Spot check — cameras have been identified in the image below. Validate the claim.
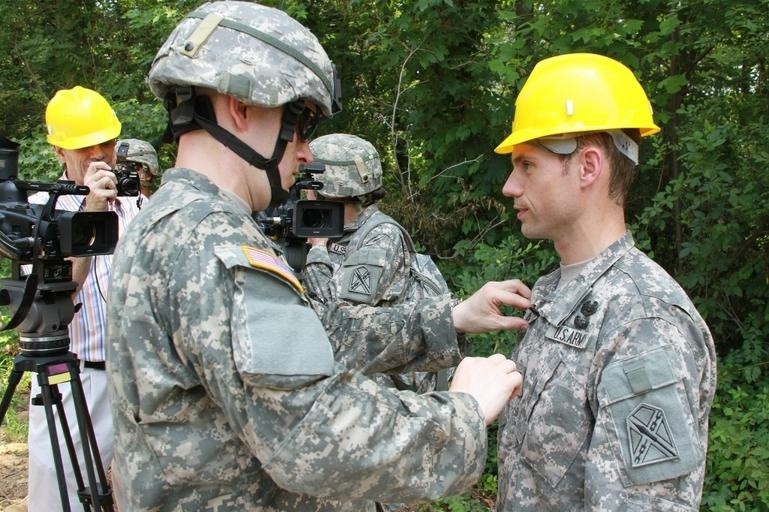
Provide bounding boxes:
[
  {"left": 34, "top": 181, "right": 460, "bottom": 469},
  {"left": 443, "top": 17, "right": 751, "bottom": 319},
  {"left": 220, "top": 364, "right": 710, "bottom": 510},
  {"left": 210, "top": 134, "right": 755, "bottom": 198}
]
[{"left": 112, "top": 170, "right": 141, "bottom": 196}]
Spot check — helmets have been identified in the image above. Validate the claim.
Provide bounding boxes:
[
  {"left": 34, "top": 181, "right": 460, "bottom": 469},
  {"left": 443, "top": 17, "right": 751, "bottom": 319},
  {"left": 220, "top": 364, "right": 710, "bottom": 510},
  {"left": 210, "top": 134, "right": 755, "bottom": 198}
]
[
  {"left": 45, "top": 86, "right": 123, "bottom": 151},
  {"left": 494, "top": 52, "right": 662, "bottom": 155},
  {"left": 148, "top": 1, "right": 335, "bottom": 121},
  {"left": 114, "top": 138, "right": 160, "bottom": 176},
  {"left": 307, "top": 133, "right": 383, "bottom": 199}
]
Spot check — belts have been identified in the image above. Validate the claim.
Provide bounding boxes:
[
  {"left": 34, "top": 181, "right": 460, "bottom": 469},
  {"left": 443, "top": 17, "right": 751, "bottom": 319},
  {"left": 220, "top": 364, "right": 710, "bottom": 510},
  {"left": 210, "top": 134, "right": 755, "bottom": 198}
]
[{"left": 84, "top": 361, "right": 105, "bottom": 368}]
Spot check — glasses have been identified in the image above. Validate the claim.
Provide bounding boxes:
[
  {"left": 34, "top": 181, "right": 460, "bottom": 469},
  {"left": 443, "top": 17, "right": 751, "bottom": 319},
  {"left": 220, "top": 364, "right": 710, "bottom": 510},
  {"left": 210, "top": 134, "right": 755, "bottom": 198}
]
[{"left": 294, "top": 106, "right": 321, "bottom": 143}]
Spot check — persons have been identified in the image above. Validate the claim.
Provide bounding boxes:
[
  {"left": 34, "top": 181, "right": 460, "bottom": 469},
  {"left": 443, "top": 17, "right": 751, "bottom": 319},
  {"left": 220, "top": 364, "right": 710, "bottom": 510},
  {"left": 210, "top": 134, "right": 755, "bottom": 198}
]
[
  {"left": 104, "top": 0, "right": 532, "bottom": 512},
  {"left": 493, "top": 52, "right": 717, "bottom": 512},
  {"left": 115, "top": 139, "right": 158, "bottom": 199},
  {"left": 28, "top": 85, "right": 149, "bottom": 512},
  {"left": 303, "top": 133, "right": 457, "bottom": 395}
]
